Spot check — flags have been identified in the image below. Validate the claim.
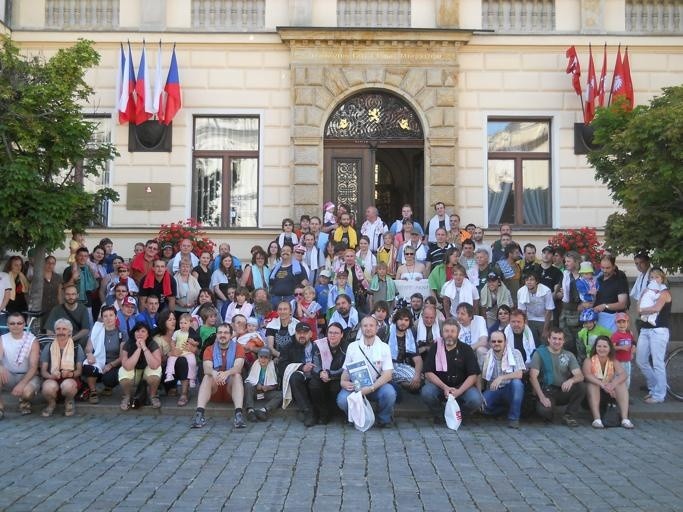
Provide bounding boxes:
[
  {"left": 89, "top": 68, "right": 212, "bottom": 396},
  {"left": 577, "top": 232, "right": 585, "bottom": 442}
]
[
  {"left": 585, "top": 46, "right": 597, "bottom": 127},
  {"left": 117, "top": 41, "right": 128, "bottom": 126},
  {"left": 566, "top": 45, "right": 581, "bottom": 95},
  {"left": 119, "top": 41, "right": 137, "bottom": 123},
  {"left": 611, "top": 47, "right": 634, "bottom": 112},
  {"left": 134, "top": 39, "right": 152, "bottom": 125},
  {"left": 597, "top": 47, "right": 610, "bottom": 107},
  {"left": 160, "top": 42, "right": 182, "bottom": 124},
  {"left": 150, "top": 39, "right": 166, "bottom": 125}
]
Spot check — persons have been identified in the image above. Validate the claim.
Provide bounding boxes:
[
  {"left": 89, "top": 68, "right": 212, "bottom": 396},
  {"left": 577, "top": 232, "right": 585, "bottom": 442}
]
[
  {"left": 575, "top": 261, "right": 603, "bottom": 303},
  {"left": 346, "top": 298, "right": 390, "bottom": 344},
  {"left": 494, "top": 234, "right": 515, "bottom": 262},
  {"left": 446, "top": 214, "right": 471, "bottom": 252},
  {"left": 280, "top": 322, "right": 317, "bottom": 426},
  {"left": 480, "top": 271, "right": 517, "bottom": 329},
  {"left": 314, "top": 270, "right": 334, "bottom": 329},
  {"left": 210, "top": 243, "right": 243, "bottom": 283},
  {"left": 98, "top": 256, "right": 124, "bottom": 304},
  {"left": 0, "top": 271, "right": 12, "bottom": 336},
  {"left": 97, "top": 282, "right": 139, "bottom": 323},
  {"left": 465, "top": 223, "right": 477, "bottom": 235},
  {"left": 40, "top": 318, "right": 86, "bottom": 417},
  {"left": 533, "top": 246, "right": 564, "bottom": 328},
  {"left": 67, "top": 232, "right": 98, "bottom": 281},
  {"left": 106, "top": 264, "right": 139, "bottom": 307},
  {"left": 640, "top": 280, "right": 661, "bottom": 326},
  {"left": 82, "top": 305, "right": 128, "bottom": 404},
  {"left": 224, "top": 287, "right": 255, "bottom": 324},
  {"left": 136, "top": 295, "right": 161, "bottom": 337},
  {"left": 611, "top": 313, "right": 636, "bottom": 406},
  {"left": 457, "top": 239, "right": 478, "bottom": 272},
  {"left": 275, "top": 218, "right": 300, "bottom": 252},
  {"left": 323, "top": 204, "right": 356, "bottom": 233},
  {"left": 517, "top": 271, "right": 555, "bottom": 349},
  {"left": 100, "top": 238, "right": 119, "bottom": 274},
  {"left": 494, "top": 243, "right": 521, "bottom": 309},
  {"left": 173, "top": 256, "right": 202, "bottom": 331},
  {"left": 270, "top": 244, "right": 309, "bottom": 311},
  {"left": 408, "top": 304, "right": 443, "bottom": 394},
  {"left": 577, "top": 308, "right": 613, "bottom": 358},
  {"left": 361, "top": 206, "right": 388, "bottom": 259},
  {"left": 243, "top": 349, "right": 283, "bottom": 422},
  {"left": 630, "top": 254, "right": 653, "bottom": 392},
  {"left": 251, "top": 245, "right": 264, "bottom": 255},
  {"left": 190, "top": 323, "right": 246, "bottom": 428},
  {"left": 491, "top": 223, "right": 523, "bottom": 264},
  {"left": 324, "top": 240, "right": 337, "bottom": 272},
  {"left": 331, "top": 242, "right": 351, "bottom": 274},
  {"left": 192, "top": 252, "right": 212, "bottom": 289},
  {"left": 191, "top": 302, "right": 220, "bottom": 331},
  {"left": 231, "top": 315, "right": 269, "bottom": 353},
  {"left": 393, "top": 217, "right": 424, "bottom": 274},
  {"left": 425, "top": 296, "right": 447, "bottom": 320},
  {"left": 323, "top": 202, "right": 337, "bottom": 237},
  {"left": 515, "top": 243, "right": 544, "bottom": 275},
  {"left": 578, "top": 254, "right": 631, "bottom": 332},
  {"left": 160, "top": 243, "right": 176, "bottom": 265},
  {"left": 457, "top": 302, "right": 488, "bottom": 393},
  {"left": 329, "top": 212, "right": 362, "bottom": 254},
  {"left": 2, "top": 256, "right": 29, "bottom": 331},
  {"left": 237, "top": 317, "right": 264, "bottom": 378},
  {"left": 328, "top": 271, "right": 356, "bottom": 322},
  {"left": 636, "top": 267, "right": 672, "bottom": 403},
  {"left": 354, "top": 235, "right": 377, "bottom": 314},
  {"left": 302, "top": 232, "right": 325, "bottom": 289},
  {"left": 164, "top": 313, "right": 202, "bottom": 388},
  {"left": 293, "top": 245, "right": 310, "bottom": 280},
  {"left": 422, "top": 202, "right": 452, "bottom": 249},
  {"left": 295, "top": 215, "right": 311, "bottom": 243},
  {"left": 44, "top": 285, "right": 89, "bottom": 385},
  {"left": 129, "top": 243, "right": 146, "bottom": 269},
  {"left": 376, "top": 308, "right": 423, "bottom": 394},
  {"left": 472, "top": 227, "right": 493, "bottom": 264},
  {"left": 465, "top": 249, "right": 511, "bottom": 317},
  {"left": 405, "top": 293, "right": 425, "bottom": 333},
  {"left": 366, "top": 261, "right": 399, "bottom": 318},
  {"left": 88, "top": 245, "right": 107, "bottom": 323},
  {"left": 117, "top": 295, "right": 136, "bottom": 343},
  {"left": 425, "top": 227, "right": 456, "bottom": 278},
  {"left": 428, "top": 247, "right": 469, "bottom": 319},
  {"left": 336, "top": 316, "right": 397, "bottom": 428},
  {"left": 153, "top": 311, "right": 200, "bottom": 406},
  {"left": 529, "top": 328, "right": 586, "bottom": 427},
  {"left": 298, "top": 286, "right": 322, "bottom": 342},
  {"left": 440, "top": 265, "right": 480, "bottom": 322},
  {"left": 0, "top": 312, "right": 40, "bottom": 420},
  {"left": 221, "top": 285, "right": 238, "bottom": 322},
  {"left": 265, "top": 300, "right": 301, "bottom": 390},
  {"left": 552, "top": 250, "right": 583, "bottom": 359},
  {"left": 328, "top": 294, "right": 369, "bottom": 336},
  {"left": 487, "top": 304, "right": 512, "bottom": 342},
  {"left": 582, "top": 336, "right": 635, "bottom": 428},
  {"left": 251, "top": 288, "right": 272, "bottom": 348},
  {"left": 390, "top": 203, "right": 424, "bottom": 240},
  {"left": 41, "top": 256, "right": 62, "bottom": 334},
  {"left": 289, "top": 287, "right": 322, "bottom": 341},
  {"left": 395, "top": 246, "right": 427, "bottom": 281},
  {"left": 138, "top": 258, "right": 177, "bottom": 313},
  {"left": 421, "top": 319, "right": 482, "bottom": 424},
  {"left": 502, "top": 309, "right": 542, "bottom": 411},
  {"left": 310, "top": 216, "right": 333, "bottom": 258},
  {"left": 264, "top": 241, "right": 282, "bottom": 272},
  {"left": 165, "top": 239, "right": 200, "bottom": 277},
  {"left": 309, "top": 322, "right": 350, "bottom": 424},
  {"left": 336, "top": 249, "right": 369, "bottom": 309},
  {"left": 189, "top": 288, "right": 224, "bottom": 328},
  {"left": 118, "top": 321, "right": 162, "bottom": 410},
  {"left": 396, "top": 228, "right": 429, "bottom": 272},
  {"left": 24, "top": 248, "right": 36, "bottom": 312},
  {"left": 63, "top": 247, "right": 99, "bottom": 341},
  {"left": 209, "top": 252, "right": 237, "bottom": 315},
  {"left": 240, "top": 251, "right": 271, "bottom": 293},
  {"left": 377, "top": 232, "right": 398, "bottom": 276},
  {"left": 195, "top": 307, "right": 219, "bottom": 384},
  {"left": 132, "top": 240, "right": 160, "bottom": 288},
  {"left": 474, "top": 330, "right": 526, "bottom": 429},
  {"left": 255, "top": 300, "right": 280, "bottom": 325}
]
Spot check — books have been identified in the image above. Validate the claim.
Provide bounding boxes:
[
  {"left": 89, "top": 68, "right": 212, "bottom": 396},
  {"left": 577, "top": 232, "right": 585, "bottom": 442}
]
[{"left": 346, "top": 360, "right": 374, "bottom": 393}]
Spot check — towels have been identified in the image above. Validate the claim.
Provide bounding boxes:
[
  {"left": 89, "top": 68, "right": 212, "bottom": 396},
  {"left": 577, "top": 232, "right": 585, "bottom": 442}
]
[
  {"left": 270, "top": 257, "right": 301, "bottom": 279},
  {"left": 396, "top": 219, "right": 403, "bottom": 233},
  {"left": 142, "top": 309, "right": 154, "bottom": 329},
  {"left": 562, "top": 270, "right": 570, "bottom": 303},
  {"left": 361, "top": 217, "right": 384, "bottom": 235},
  {"left": 346, "top": 391, "right": 365, "bottom": 428},
  {"left": 481, "top": 344, "right": 516, "bottom": 382},
  {"left": 266, "top": 315, "right": 301, "bottom": 336},
  {"left": 325, "top": 255, "right": 331, "bottom": 270},
  {"left": 519, "top": 257, "right": 542, "bottom": 270},
  {"left": 244, "top": 359, "right": 278, "bottom": 386},
  {"left": 224, "top": 301, "right": 252, "bottom": 324},
  {"left": 313, "top": 337, "right": 333, "bottom": 371},
  {"left": 317, "top": 232, "right": 329, "bottom": 252},
  {"left": 416, "top": 312, "right": 441, "bottom": 342},
  {"left": 50, "top": 338, "right": 74, "bottom": 376},
  {"left": 591, "top": 354, "right": 614, "bottom": 385},
  {"left": 143, "top": 268, "right": 172, "bottom": 296},
  {"left": 213, "top": 255, "right": 240, "bottom": 271},
  {"left": 359, "top": 334, "right": 384, "bottom": 361},
  {"left": 377, "top": 244, "right": 397, "bottom": 276},
  {"left": 174, "top": 271, "right": 202, "bottom": 301},
  {"left": 504, "top": 323, "right": 536, "bottom": 364},
  {"left": 78, "top": 265, "right": 99, "bottom": 304},
  {"left": 16, "top": 331, "right": 34, "bottom": 366},
  {"left": 187, "top": 327, "right": 202, "bottom": 349},
  {"left": 435, "top": 337, "right": 447, "bottom": 372},
  {"left": 213, "top": 337, "right": 237, "bottom": 383},
  {"left": 467, "top": 264, "right": 479, "bottom": 286},
  {"left": 87, "top": 261, "right": 107, "bottom": 279},
  {"left": 428, "top": 213, "right": 451, "bottom": 244},
  {"left": 209, "top": 268, "right": 228, "bottom": 292},
  {"left": 444, "top": 278, "right": 473, "bottom": 306},
  {"left": 303, "top": 301, "right": 322, "bottom": 318},
  {"left": 629, "top": 266, "right": 651, "bottom": 300},
  {"left": 428, "top": 264, "right": 446, "bottom": 295},
  {"left": 481, "top": 281, "right": 509, "bottom": 309},
  {"left": 328, "top": 306, "right": 358, "bottom": 330},
  {"left": 327, "top": 282, "right": 355, "bottom": 309},
  {"left": 9, "top": 272, "right": 29, "bottom": 301},
  {"left": 133, "top": 251, "right": 159, "bottom": 275},
  {"left": 332, "top": 256, "right": 340, "bottom": 273},
  {"left": 237, "top": 332, "right": 266, "bottom": 346},
  {"left": 488, "top": 319, "right": 499, "bottom": 341},
  {"left": 471, "top": 319, "right": 479, "bottom": 344},
  {"left": 311, "top": 246, "right": 318, "bottom": 270},
  {"left": 173, "top": 251, "right": 199, "bottom": 273},
  {"left": 289, "top": 299, "right": 297, "bottom": 316},
  {"left": 370, "top": 273, "right": 395, "bottom": 302},
  {"left": 251, "top": 264, "right": 270, "bottom": 291},
  {"left": 460, "top": 229, "right": 472, "bottom": 242},
  {"left": 388, "top": 322, "right": 416, "bottom": 361},
  {"left": 117, "top": 310, "right": 135, "bottom": 332},
  {"left": 279, "top": 232, "right": 298, "bottom": 248},
  {"left": 282, "top": 362, "right": 303, "bottom": 410},
  {"left": 339, "top": 263, "right": 369, "bottom": 290},
  {"left": 333, "top": 226, "right": 357, "bottom": 249},
  {"left": 83, "top": 322, "right": 106, "bottom": 373},
  {"left": 460, "top": 256, "right": 469, "bottom": 270},
  {"left": 496, "top": 259, "right": 516, "bottom": 279},
  {"left": 355, "top": 249, "right": 372, "bottom": 274},
  {"left": 519, "top": 283, "right": 547, "bottom": 304},
  {"left": 530, "top": 344, "right": 554, "bottom": 385},
  {"left": 402, "top": 238, "right": 426, "bottom": 264}
]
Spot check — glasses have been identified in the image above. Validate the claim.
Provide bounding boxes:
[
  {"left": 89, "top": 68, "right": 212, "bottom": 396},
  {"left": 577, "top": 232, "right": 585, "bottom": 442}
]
[
  {"left": 411, "top": 233, "right": 419, "bottom": 236},
  {"left": 116, "top": 290, "right": 128, "bottom": 292},
  {"left": 492, "top": 340, "right": 503, "bottom": 343},
  {"left": 404, "top": 252, "right": 414, "bottom": 255},
  {"left": 294, "top": 293, "right": 302, "bottom": 297},
  {"left": 217, "top": 331, "right": 231, "bottom": 334},
  {"left": 284, "top": 223, "right": 292, "bottom": 226},
  {"left": 100, "top": 305, "right": 113, "bottom": 310},
  {"left": 10, "top": 321, "right": 24, "bottom": 325},
  {"left": 296, "top": 250, "right": 304, "bottom": 255},
  {"left": 47, "top": 262, "right": 55, "bottom": 265},
  {"left": 148, "top": 247, "right": 158, "bottom": 250},
  {"left": 498, "top": 312, "right": 509, "bottom": 315},
  {"left": 119, "top": 270, "right": 127, "bottom": 273},
  {"left": 566, "top": 253, "right": 575, "bottom": 257}
]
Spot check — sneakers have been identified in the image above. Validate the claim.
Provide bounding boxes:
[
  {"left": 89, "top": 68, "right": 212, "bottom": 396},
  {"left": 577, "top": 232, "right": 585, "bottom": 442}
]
[
  {"left": 190, "top": 411, "right": 207, "bottom": 428},
  {"left": 233, "top": 412, "right": 247, "bottom": 428},
  {"left": 296, "top": 410, "right": 305, "bottom": 423},
  {"left": 101, "top": 387, "right": 112, "bottom": 396},
  {"left": 508, "top": 419, "right": 520, "bottom": 429},
  {"left": 621, "top": 422, "right": 634, "bottom": 429},
  {"left": 168, "top": 391, "right": 178, "bottom": 397},
  {"left": 377, "top": 422, "right": 393, "bottom": 428},
  {"left": 164, "top": 375, "right": 174, "bottom": 382},
  {"left": 544, "top": 413, "right": 554, "bottom": 424},
  {"left": 496, "top": 414, "right": 506, "bottom": 420},
  {"left": 149, "top": 395, "right": 161, "bottom": 409},
  {"left": 246, "top": 411, "right": 258, "bottom": 422},
  {"left": 317, "top": 416, "right": 331, "bottom": 425},
  {"left": 561, "top": 414, "right": 578, "bottom": 427},
  {"left": 304, "top": 415, "right": 317, "bottom": 427},
  {"left": 434, "top": 416, "right": 446, "bottom": 425},
  {"left": 120, "top": 396, "right": 130, "bottom": 410},
  {"left": 89, "top": 391, "right": 99, "bottom": 404},
  {"left": 592, "top": 421, "right": 604, "bottom": 429},
  {"left": 256, "top": 409, "right": 268, "bottom": 422},
  {"left": 190, "top": 381, "right": 196, "bottom": 388},
  {"left": 177, "top": 398, "right": 189, "bottom": 406}
]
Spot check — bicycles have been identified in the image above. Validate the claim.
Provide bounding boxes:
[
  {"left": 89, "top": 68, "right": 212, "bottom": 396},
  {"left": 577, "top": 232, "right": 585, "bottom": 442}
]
[
  {"left": 1, "top": 311, "right": 83, "bottom": 387},
  {"left": 666, "top": 345, "right": 683, "bottom": 402}
]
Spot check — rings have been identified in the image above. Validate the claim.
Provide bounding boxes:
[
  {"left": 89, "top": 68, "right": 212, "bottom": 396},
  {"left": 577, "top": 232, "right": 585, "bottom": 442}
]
[{"left": 89, "top": 360, "right": 90, "bottom": 362}]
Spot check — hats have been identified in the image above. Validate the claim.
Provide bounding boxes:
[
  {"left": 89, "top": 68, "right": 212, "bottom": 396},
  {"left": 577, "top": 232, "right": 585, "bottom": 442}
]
[
  {"left": 486, "top": 271, "right": 498, "bottom": 282},
  {"left": 163, "top": 242, "right": 173, "bottom": 249},
  {"left": 294, "top": 245, "right": 306, "bottom": 252},
  {"left": 615, "top": 312, "right": 629, "bottom": 322},
  {"left": 410, "top": 228, "right": 421, "bottom": 235},
  {"left": 323, "top": 202, "right": 335, "bottom": 210},
  {"left": 258, "top": 347, "right": 272, "bottom": 359},
  {"left": 578, "top": 261, "right": 594, "bottom": 273},
  {"left": 295, "top": 322, "right": 312, "bottom": 332},
  {"left": 410, "top": 293, "right": 423, "bottom": 299},
  {"left": 116, "top": 263, "right": 130, "bottom": 269},
  {"left": 247, "top": 317, "right": 258, "bottom": 326},
  {"left": 647, "top": 280, "right": 660, "bottom": 291},
  {"left": 121, "top": 297, "right": 137, "bottom": 306},
  {"left": 542, "top": 246, "right": 555, "bottom": 255},
  {"left": 318, "top": 269, "right": 332, "bottom": 278}
]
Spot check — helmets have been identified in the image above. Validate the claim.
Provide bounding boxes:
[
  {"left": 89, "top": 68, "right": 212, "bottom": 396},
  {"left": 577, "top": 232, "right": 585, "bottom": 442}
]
[{"left": 580, "top": 308, "right": 598, "bottom": 321}]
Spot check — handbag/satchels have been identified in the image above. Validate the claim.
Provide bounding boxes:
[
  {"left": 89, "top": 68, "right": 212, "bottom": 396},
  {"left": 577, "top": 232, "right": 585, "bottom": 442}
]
[
  {"left": 358, "top": 345, "right": 404, "bottom": 404},
  {"left": 74, "top": 340, "right": 90, "bottom": 402},
  {"left": 563, "top": 314, "right": 580, "bottom": 327},
  {"left": 129, "top": 368, "right": 147, "bottom": 410}
]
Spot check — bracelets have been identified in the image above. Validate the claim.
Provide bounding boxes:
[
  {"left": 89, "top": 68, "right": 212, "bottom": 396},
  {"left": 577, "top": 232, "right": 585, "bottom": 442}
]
[
  {"left": 72, "top": 372, "right": 74, "bottom": 377},
  {"left": 86, "top": 354, "right": 92, "bottom": 355},
  {"left": 440, "top": 384, "right": 445, "bottom": 388},
  {"left": 71, "top": 277, "right": 76, "bottom": 281},
  {"left": 143, "top": 347, "right": 148, "bottom": 352},
  {"left": 369, "top": 386, "right": 375, "bottom": 392},
  {"left": 109, "top": 363, "right": 114, "bottom": 368}
]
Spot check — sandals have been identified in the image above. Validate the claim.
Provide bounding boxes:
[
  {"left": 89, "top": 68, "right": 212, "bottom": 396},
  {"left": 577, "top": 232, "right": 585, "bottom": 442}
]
[
  {"left": 645, "top": 398, "right": 659, "bottom": 403},
  {"left": 0, "top": 408, "right": 5, "bottom": 421},
  {"left": 644, "top": 395, "right": 651, "bottom": 400},
  {"left": 19, "top": 400, "right": 32, "bottom": 416},
  {"left": 41, "top": 405, "right": 56, "bottom": 418},
  {"left": 64, "top": 399, "right": 76, "bottom": 417}
]
